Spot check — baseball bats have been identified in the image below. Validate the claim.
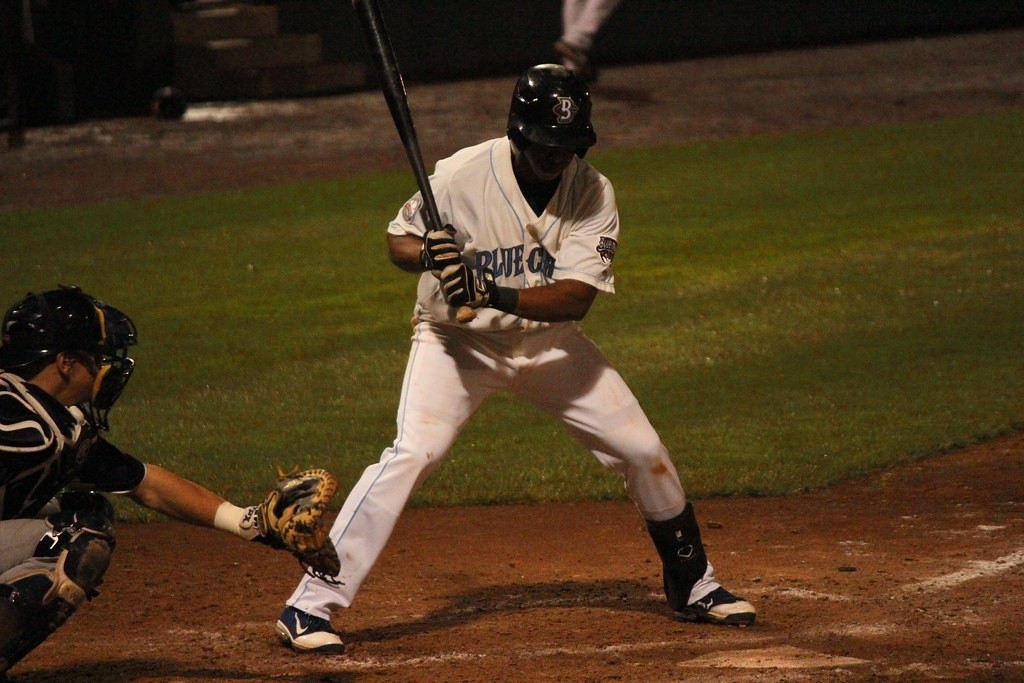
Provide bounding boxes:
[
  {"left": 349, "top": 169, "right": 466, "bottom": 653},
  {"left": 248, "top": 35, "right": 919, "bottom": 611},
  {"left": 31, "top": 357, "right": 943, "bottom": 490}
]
[{"left": 358, "top": 0, "right": 476, "bottom": 325}]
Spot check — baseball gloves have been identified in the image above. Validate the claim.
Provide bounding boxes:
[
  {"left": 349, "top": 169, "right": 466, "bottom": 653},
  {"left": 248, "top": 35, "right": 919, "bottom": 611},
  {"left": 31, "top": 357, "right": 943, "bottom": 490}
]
[{"left": 256, "top": 470, "right": 341, "bottom": 576}]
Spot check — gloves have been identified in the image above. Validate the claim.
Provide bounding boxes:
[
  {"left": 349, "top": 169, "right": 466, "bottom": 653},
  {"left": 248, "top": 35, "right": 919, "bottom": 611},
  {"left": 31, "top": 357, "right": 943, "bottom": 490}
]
[
  {"left": 431, "top": 264, "right": 498, "bottom": 309},
  {"left": 419, "top": 224, "right": 461, "bottom": 268}
]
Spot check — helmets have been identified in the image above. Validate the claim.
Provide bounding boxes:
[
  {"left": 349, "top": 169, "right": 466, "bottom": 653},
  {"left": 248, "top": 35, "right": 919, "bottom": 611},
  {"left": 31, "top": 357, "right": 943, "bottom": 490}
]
[
  {"left": 506, "top": 64, "right": 598, "bottom": 160},
  {"left": 0, "top": 283, "right": 138, "bottom": 373}
]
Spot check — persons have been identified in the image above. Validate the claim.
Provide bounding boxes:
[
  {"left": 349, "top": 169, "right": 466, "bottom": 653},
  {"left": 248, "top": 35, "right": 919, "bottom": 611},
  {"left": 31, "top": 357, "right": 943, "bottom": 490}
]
[
  {"left": 271, "top": 64, "right": 760, "bottom": 655},
  {"left": 0, "top": 284, "right": 344, "bottom": 682}
]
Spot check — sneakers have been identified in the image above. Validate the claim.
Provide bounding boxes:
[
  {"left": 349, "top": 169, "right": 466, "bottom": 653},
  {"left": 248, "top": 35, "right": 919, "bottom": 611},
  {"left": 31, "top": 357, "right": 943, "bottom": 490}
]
[
  {"left": 672, "top": 586, "right": 757, "bottom": 624},
  {"left": 276, "top": 605, "right": 345, "bottom": 654}
]
